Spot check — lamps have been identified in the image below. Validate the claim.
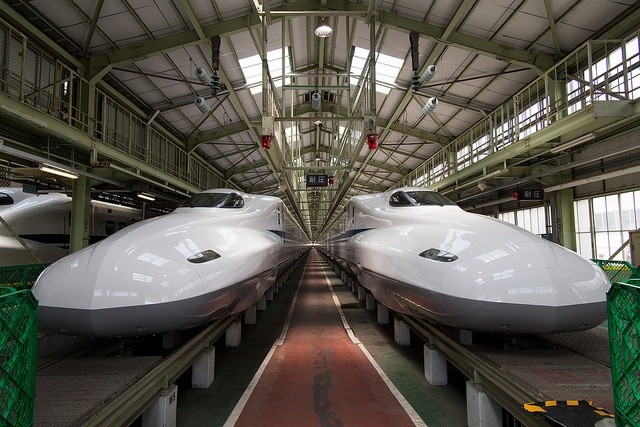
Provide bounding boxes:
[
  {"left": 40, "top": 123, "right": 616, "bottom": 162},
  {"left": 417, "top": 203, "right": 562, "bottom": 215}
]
[{"left": 314, "top": 19, "right": 334, "bottom": 39}]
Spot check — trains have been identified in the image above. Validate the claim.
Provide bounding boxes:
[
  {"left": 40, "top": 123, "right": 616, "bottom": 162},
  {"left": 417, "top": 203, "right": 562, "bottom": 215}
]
[
  {"left": 31, "top": 187, "right": 313, "bottom": 336},
  {"left": 0, "top": 181, "right": 168, "bottom": 267},
  {"left": 313, "top": 187, "right": 612, "bottom": 337}
]
